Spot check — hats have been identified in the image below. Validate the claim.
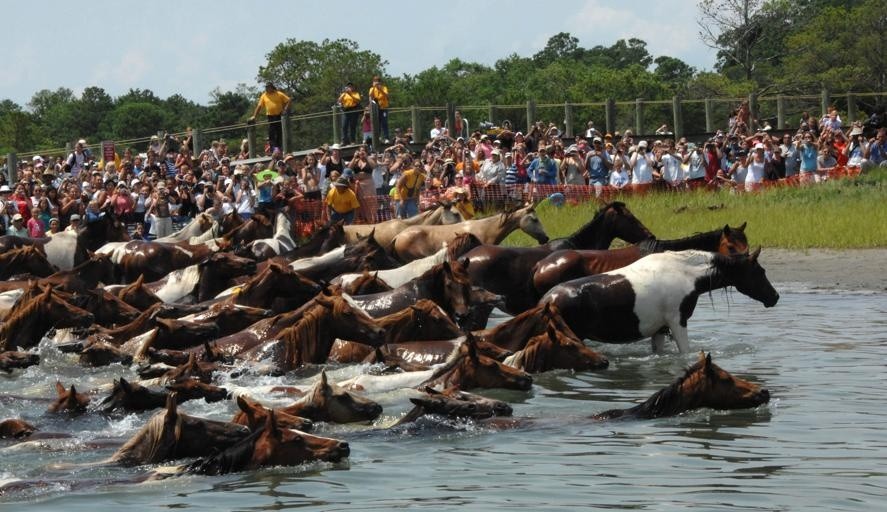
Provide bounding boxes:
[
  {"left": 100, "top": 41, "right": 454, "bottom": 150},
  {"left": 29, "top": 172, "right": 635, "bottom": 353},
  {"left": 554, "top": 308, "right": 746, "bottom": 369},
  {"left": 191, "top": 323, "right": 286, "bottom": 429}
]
[
  {"left": 0, "top": 128, "right": 387, "bottom": 228},
  {"left": 444, "top": 112, "right": 877, "bottom": 184}
]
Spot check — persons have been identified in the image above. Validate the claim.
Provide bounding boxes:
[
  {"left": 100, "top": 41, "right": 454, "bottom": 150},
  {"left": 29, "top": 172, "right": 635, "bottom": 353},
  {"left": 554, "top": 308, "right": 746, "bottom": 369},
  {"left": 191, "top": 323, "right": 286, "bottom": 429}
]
[
  {"left": 337, "top": 82, "right": 362, "bottom": 146},
  {"left": 466, "top": 106, "right": 887, "bottom": 206},
  {"left": 368, "top": 76, "right": 390, "bottom": 145},
  {"left": 247, "top": 82, "right": 292, "bottom": 154},
  {"left": 453, "top": 110, "right": 470, "bottom": 137},
  {"left": 1, "top": 119, "right": 477, "bottom": 240}
]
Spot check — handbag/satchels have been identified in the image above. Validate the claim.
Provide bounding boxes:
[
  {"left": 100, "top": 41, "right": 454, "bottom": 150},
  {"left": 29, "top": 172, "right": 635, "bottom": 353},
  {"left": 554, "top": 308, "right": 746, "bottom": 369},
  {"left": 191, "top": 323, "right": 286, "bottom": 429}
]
[{"left": 407, "top": 187, "right": 414, "bottom": 196}]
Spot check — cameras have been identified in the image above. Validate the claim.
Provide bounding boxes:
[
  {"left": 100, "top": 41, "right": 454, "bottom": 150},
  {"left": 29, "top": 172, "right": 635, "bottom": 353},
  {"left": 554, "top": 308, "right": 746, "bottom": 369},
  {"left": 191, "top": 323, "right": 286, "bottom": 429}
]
[
  {"left": 76, "top": 199, "right": 81, "bottom": 204},
  {"left": 120, "top": 188, "right": 125, "bottom": 193},
  {"left": 355, "top": 151, "right": 363, "bottom": 156},
  {"left": 801, "top": 140, "right": 810, "bottom": 144},
  {"left": 306, "top": 166, "right": 311, "bottom": 170},
  {"left": 751, "top": 148, "right": 756, "bottom": 153}
]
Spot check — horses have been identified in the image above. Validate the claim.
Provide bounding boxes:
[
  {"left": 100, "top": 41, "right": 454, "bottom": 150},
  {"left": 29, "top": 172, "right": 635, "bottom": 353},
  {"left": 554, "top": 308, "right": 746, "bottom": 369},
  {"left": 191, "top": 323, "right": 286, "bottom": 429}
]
[{"left": 0, "top": 198, "right": 781, "bottom": 495}]
